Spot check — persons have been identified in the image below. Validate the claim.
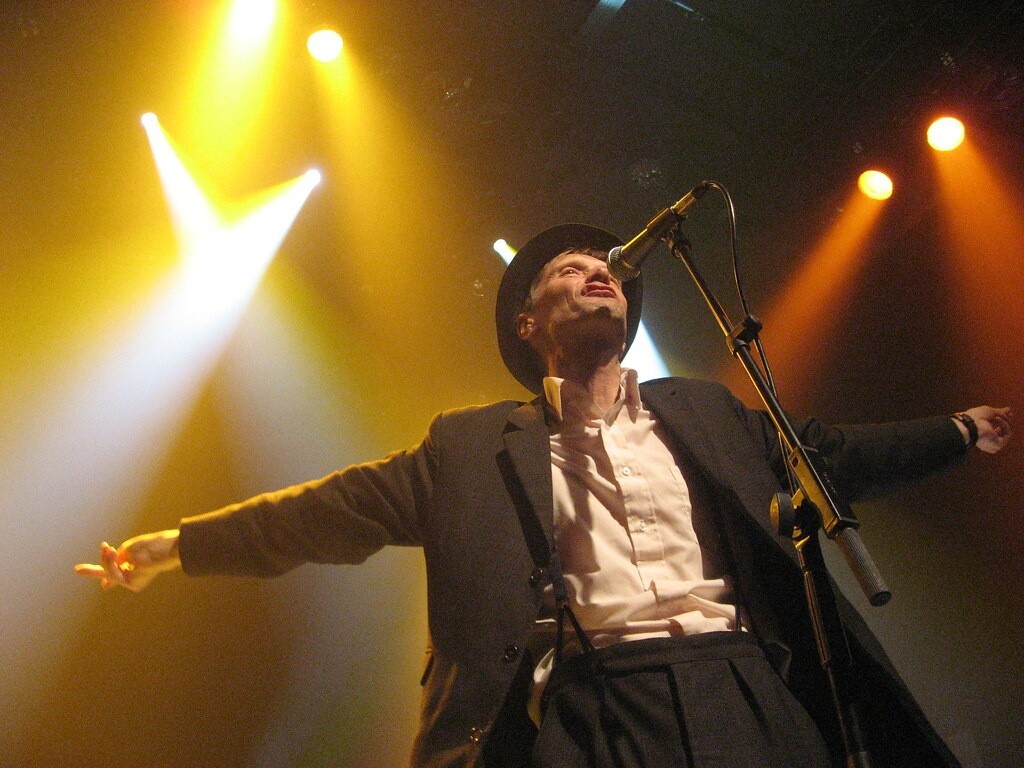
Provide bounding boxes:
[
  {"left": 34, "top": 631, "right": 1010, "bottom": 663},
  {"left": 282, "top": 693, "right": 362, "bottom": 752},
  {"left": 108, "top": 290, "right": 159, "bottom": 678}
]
[{"left": 74, "top": 224, "right": 1024, "bottom": 768}]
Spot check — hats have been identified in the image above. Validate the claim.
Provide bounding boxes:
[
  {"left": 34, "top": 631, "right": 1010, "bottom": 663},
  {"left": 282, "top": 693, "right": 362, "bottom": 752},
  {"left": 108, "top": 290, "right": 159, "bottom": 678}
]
[{"left": 495, "top": 221, "right": 643, "bottom": 396}]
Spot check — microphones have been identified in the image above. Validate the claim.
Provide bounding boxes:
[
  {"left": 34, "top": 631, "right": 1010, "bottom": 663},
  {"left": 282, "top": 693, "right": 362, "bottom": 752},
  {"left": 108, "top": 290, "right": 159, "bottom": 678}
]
[{"left": 606, "top": 178, "right": 712, "bottom": 282}]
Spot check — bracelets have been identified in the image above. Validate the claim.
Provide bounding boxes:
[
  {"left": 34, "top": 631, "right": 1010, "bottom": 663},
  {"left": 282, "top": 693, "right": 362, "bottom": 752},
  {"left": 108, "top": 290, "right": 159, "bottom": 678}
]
[{"left": 949, "top": 413, "right": 979, "bottom": 450}]
